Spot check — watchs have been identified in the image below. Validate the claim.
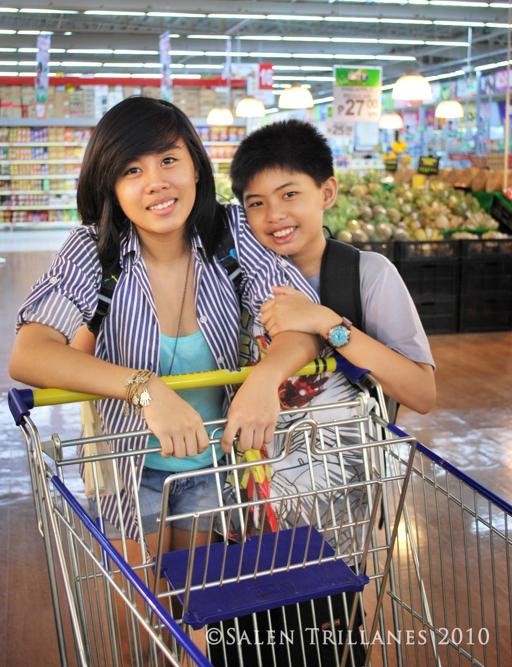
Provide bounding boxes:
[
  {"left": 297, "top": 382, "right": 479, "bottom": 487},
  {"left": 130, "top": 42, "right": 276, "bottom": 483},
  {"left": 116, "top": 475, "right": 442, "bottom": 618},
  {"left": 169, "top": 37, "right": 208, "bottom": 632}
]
[{"left": 327, "top": 313, "right": 353, "bottom": 352}]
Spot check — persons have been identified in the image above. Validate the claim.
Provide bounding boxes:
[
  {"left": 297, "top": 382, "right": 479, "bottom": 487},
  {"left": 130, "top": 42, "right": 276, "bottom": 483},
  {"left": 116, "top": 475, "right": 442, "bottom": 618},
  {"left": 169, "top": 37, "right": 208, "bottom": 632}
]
[
  {"left": 9, "top": 92, "right": 320, "bottom": 666},
  {"left": 69, "top": 116, "right": 439, "bottom": 666}
]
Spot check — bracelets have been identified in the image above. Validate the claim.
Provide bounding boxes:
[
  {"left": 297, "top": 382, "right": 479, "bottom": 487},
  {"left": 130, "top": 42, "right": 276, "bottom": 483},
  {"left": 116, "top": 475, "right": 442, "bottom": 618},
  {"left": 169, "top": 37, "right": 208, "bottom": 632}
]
[{"left": 123, "top": 368, "right": 154, "bottom": 412}]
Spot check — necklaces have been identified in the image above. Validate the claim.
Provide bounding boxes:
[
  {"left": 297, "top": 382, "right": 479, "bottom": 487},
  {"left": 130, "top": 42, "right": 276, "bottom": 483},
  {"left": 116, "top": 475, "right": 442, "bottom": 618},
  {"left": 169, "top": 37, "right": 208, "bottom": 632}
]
[{"left": 160, "top": 251, "right": 191, "bottom": 379}]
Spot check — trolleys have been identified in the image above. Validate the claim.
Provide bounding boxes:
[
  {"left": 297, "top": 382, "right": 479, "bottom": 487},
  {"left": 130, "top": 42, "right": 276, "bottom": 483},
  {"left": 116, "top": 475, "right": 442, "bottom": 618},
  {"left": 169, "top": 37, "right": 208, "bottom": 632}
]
[{"left": 7, "top": 356, "right": 512, "bottom": 667}]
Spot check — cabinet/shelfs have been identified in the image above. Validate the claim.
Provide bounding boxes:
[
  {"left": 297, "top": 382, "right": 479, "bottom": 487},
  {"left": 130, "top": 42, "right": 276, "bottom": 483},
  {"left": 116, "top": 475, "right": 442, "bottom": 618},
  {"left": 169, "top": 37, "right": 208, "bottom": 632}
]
[{"left": 1, "top": 77, "right": 249, "bottom": 253}]
